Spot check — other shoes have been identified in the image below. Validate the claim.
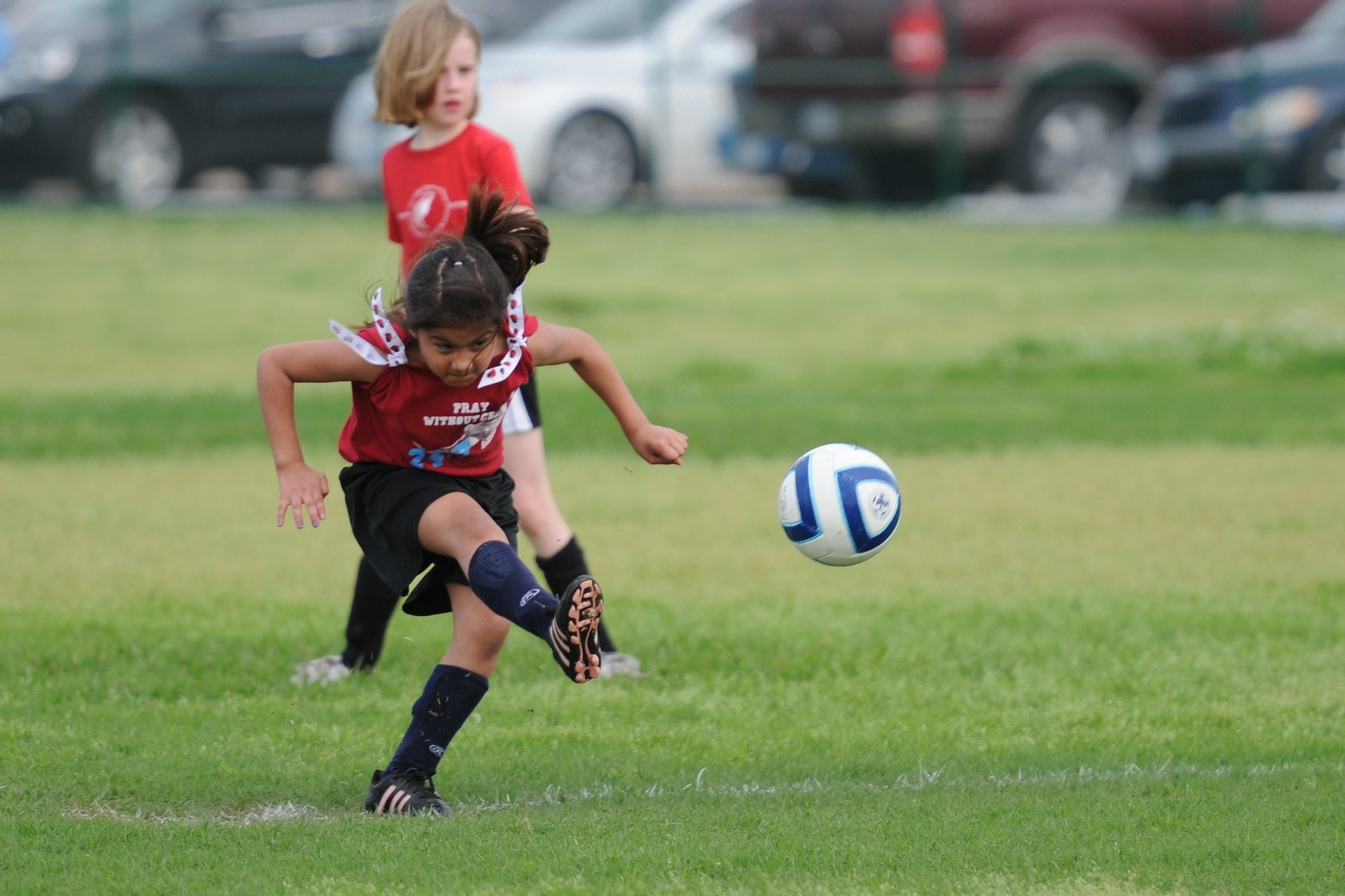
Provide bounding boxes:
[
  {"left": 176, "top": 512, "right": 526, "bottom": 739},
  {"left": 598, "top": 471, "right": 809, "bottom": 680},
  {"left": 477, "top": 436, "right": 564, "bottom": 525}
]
[
  {"left": 598, "top": 652, "right": 641, "bottom": 680},
  {"left": 290, "top": 654, "right": 353, "bottom": 684}
]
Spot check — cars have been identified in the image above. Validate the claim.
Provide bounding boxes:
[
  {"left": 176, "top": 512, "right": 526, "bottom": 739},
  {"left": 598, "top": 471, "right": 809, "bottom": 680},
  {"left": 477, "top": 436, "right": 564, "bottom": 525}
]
[
  {"left": 322, "top": 0, "right": 784, "bottom": 221},
  {"left": 728, "top": 0, "right": 1345, "bottom": 235},
  {"left": 0, "top": 0, "right": 569, "bottom": 218}
]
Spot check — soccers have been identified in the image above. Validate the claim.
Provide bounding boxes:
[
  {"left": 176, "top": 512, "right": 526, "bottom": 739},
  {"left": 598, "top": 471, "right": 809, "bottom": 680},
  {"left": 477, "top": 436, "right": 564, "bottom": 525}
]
[{"left": 774, "top": 443, "right": 901, "bottom": 568}]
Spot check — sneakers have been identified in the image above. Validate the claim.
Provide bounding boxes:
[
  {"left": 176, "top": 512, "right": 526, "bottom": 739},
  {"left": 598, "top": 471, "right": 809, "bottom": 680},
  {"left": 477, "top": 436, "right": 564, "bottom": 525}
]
[
  {"left": 549, "top": 575, "right": 602, "bottom": 684},
  {"left": 363, "top": 768, "right": 447, "bottom": 819}
]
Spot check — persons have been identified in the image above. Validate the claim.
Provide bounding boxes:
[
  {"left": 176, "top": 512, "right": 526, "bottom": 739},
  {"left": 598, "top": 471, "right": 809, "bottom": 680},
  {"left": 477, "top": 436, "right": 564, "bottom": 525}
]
[
  {"left": 255, "top": 181, "right": 688, "bottom": 818},
  {"left": 374, "top": 0, "right": 643, "bottom": 677}
]
